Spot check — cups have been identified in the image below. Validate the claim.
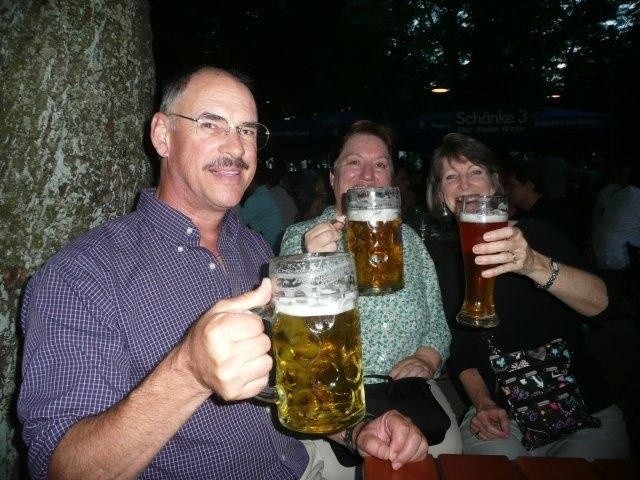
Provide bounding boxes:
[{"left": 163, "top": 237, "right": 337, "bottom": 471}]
[
  {"left": 329, "top": 187, "right": 405, "bottom": 297},
  {"left": 453, "top": 194, "right": 509, "bottom": 330},
  {"left": 248, "top": 251, "right": 367, "bottom": 434}
]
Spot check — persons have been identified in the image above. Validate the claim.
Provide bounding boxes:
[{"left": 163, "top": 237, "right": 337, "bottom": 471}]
[
  {"left": 239, "top": 159, "right": 331, "bottom": 256},
  {"left": 14, "top": 65, "right": 427, "bottom": 480},
  {"left": 505, "top": 160, "right": 638, "bottom": 306},
  {"left": 277, "top": 120, "right": 463, "bottom": 458},
  {"left": 424, "top": 131, "right": 631, "bottom": 459},
  {"left": 391, "top": 165, "right": 459, "bottom": 237}
]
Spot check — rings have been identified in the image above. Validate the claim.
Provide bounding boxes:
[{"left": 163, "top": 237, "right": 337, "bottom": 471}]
[{"left": 476, "top": 431, "right": 480, "bottom": 435}]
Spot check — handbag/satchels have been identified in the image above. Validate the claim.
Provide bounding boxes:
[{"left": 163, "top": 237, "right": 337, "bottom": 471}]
[
  {"left": 326, "top": 374, "right": 452, "bottom": 468},
  {"left": 487, "top": 335, "right": 601, "bottom": 452}
]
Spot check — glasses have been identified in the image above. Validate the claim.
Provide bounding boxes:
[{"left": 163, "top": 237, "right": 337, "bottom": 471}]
[{"left": 168, "top": 111, "right": 271, "bottom": 152}]
